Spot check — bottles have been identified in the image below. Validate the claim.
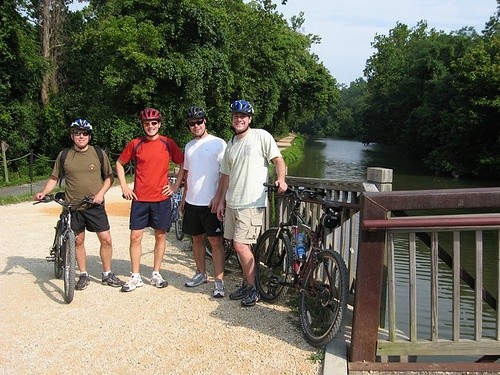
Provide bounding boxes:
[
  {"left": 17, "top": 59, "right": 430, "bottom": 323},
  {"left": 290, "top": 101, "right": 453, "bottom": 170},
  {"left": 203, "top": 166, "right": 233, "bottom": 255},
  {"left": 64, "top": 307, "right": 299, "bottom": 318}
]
[
  {"left": 178, "top": 193, "right": 181, "bottom": 200},
  {"left": 297, "top": 234, "right": 305, "bottom": 259}
]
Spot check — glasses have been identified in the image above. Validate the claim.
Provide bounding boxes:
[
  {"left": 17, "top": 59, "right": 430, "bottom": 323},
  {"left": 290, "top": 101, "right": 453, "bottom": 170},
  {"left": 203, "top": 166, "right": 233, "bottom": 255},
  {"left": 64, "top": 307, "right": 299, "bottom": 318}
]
[
  {"left": 72, "top": 131, "right": 90, "bottom": 136},
  {"left": 143, "top": 122, "right": 159, "bottom": 126},
  {"left": 187, "top": 119, "right": 204, "bottom": 126}
]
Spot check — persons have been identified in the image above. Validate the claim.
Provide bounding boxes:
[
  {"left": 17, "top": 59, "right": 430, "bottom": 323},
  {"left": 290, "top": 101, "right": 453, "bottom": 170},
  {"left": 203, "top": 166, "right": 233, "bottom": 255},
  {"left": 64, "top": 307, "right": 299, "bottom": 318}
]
[
  {"left": 217, "top": 101, "right": 287, "bottom": 305},
  {"left": 116, "top": 109, "right": 185, "bottom": 292},
  {"left": 34, "top": 119, "right": 126, "bottom": 290},
  {"left": 180, "top": 107, "right": 226, "bottom": 297}
]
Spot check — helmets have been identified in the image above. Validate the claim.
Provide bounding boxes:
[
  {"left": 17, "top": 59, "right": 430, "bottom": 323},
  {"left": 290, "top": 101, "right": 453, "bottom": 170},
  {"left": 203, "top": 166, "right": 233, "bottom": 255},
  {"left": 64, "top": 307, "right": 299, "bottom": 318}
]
[
  {"left": 139, "top": 108, "right": 162, "bottom": 122},
  {"left": 229, "top": 100, "right": 254, "bottom": 116},
  {"left": 70, "top": 119, "right": 94, "bottom": 131},
  {"left": 185, "top": 105, "right": 207, "bottom": 121}
]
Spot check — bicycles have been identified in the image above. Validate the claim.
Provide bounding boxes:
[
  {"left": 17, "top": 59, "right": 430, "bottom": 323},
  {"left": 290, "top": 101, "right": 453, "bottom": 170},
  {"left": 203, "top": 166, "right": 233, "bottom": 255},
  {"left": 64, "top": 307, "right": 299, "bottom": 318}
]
[
  {"left": 33, "top": 191, "right": 104, "bottom": 303},
  {"left": 254, "top": 183, "right": 350, "bottom": 347},
  {"left": 166, "top": 173, "right": 255, "bottom": 272}
]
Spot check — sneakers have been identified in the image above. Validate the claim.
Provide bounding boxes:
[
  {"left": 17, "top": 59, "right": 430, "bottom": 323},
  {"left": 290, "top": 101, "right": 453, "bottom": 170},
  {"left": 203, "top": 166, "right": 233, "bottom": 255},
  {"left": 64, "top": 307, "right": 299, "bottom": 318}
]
[
  {"left": 185, "top": 270, "right": 209, "bottom": 287},
  {"left": 74, "top": 272, "right": 90, "bottom": 290},
  {"left": 149, "top": 271, "right": 168, "bottom": 287},
  {"left": 229, "top": 286, "right": 254, "bottom": 300},
  {"left": 121, "top": 272, "right": 144, "bottom": 291},
  {"left": 214, "top": 278, "right": 225, "bottom": 298},
  {"left": 102, "top": 272, "right": 126, "bottom": 286},
  {"left": 241, "top": 289, "right": 261, "bottom": 306}
]
